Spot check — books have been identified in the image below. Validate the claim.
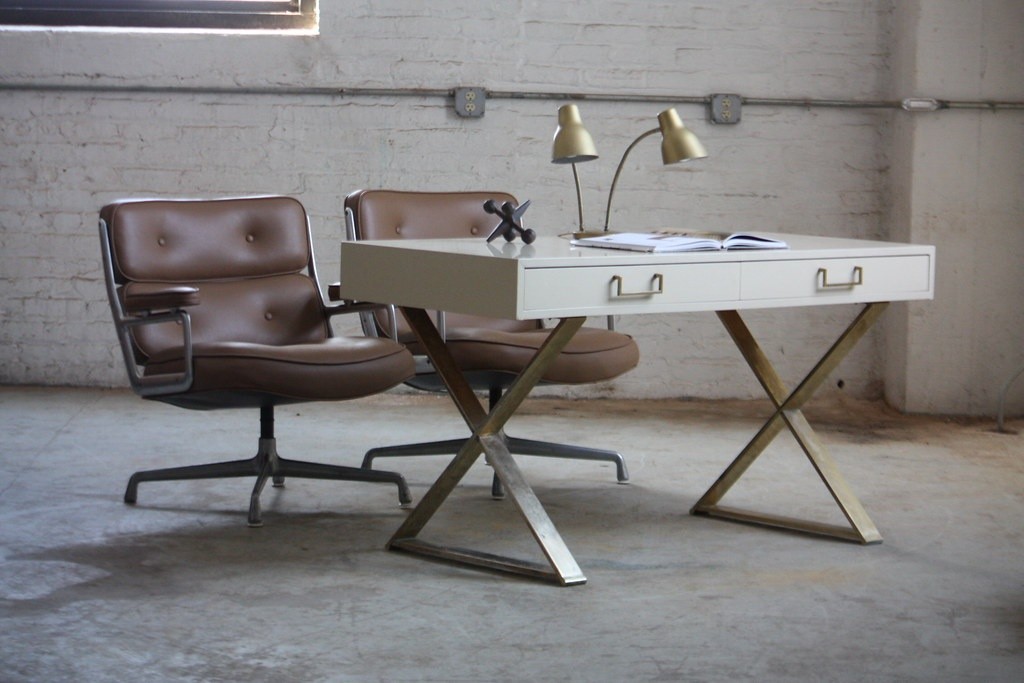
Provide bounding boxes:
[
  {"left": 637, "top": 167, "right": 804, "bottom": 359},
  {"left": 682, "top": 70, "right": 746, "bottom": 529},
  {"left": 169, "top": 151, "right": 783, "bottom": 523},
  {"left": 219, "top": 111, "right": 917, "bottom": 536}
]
[{"left": 570, "top": 231, "right": 789, "bottom": 252}]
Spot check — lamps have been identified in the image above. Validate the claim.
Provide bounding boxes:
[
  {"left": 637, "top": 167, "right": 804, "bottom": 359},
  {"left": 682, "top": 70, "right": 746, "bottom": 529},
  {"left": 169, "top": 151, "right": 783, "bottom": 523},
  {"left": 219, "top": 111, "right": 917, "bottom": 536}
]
[
  {"left": 600, "top": 108, "right": 710, "bottom": 238},
  {"left": 551, "top": 103, "right": 599, "bottom": 239}
]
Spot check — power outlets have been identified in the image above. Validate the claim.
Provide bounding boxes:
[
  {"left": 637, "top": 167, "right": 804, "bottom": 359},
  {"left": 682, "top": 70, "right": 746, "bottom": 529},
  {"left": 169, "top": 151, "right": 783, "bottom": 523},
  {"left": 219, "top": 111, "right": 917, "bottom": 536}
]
[
  {"left": 710, "top": 93, "right": 742, "bottom": 124},
  {"left": 455, "top": 87, "right": 486, "bottom": 117}
]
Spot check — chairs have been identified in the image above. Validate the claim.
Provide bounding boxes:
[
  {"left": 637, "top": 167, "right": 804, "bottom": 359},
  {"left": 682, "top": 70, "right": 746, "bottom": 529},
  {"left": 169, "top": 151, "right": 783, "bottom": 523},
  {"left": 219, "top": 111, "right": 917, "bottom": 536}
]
[
  {"left": 98, "top": 193, "right": 419, "bottom": 527},
  {"left": 346, "top": 190, "right": 641, "bottom": 500}
]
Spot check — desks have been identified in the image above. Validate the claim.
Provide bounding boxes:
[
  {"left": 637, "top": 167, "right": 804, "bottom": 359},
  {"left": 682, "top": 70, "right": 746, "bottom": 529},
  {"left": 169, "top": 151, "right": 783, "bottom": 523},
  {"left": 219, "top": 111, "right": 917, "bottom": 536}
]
[{"left": 335, "top": 233, "right": 939, "bottom": 587}]
